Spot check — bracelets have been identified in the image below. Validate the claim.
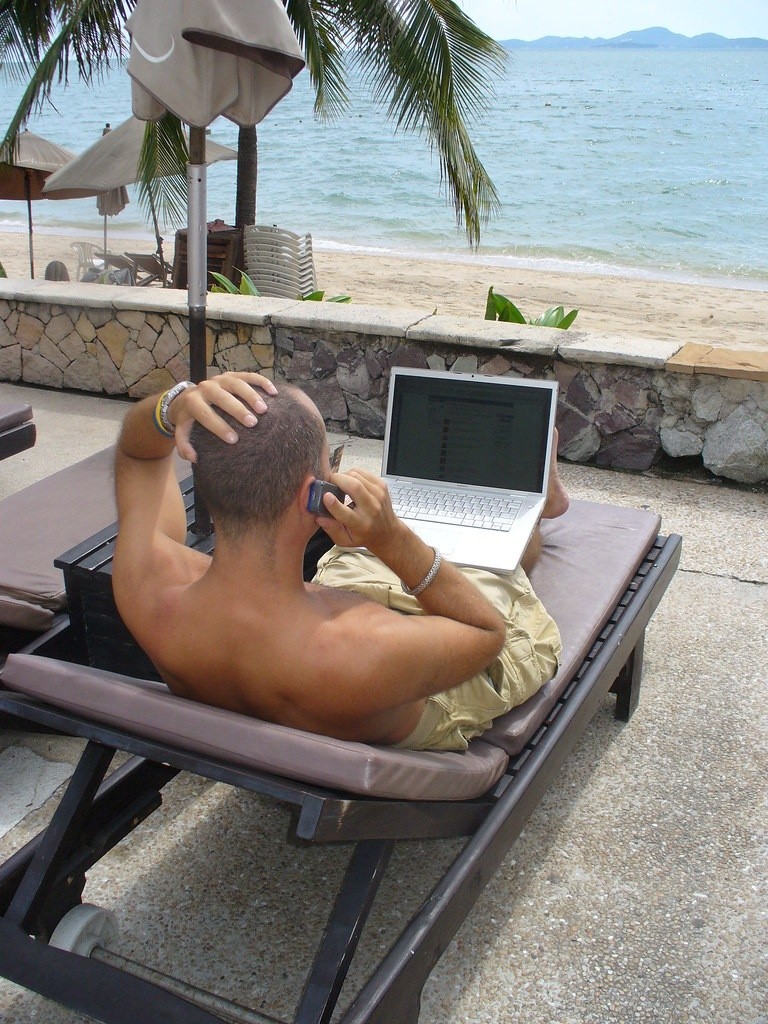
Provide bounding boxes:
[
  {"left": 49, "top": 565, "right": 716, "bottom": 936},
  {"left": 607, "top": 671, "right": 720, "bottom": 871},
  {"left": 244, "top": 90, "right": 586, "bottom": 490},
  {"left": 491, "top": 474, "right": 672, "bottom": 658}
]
[
  {"left": 403, "top": 547, "right": 441, "bottom": 595},
  {"left": 153, "top": 388, "right": 176, "bottom": 439}
]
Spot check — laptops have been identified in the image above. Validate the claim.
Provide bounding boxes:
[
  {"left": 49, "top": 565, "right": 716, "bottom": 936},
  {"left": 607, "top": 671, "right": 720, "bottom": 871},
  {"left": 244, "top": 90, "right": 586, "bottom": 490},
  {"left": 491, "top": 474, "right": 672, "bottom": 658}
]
[{"left": 379, "top": 366, "right": 558, "bottom": 575}]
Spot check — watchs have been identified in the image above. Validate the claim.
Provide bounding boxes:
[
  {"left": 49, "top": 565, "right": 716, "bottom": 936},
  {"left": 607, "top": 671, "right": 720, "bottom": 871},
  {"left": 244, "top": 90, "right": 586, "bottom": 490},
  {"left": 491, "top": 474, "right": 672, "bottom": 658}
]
[{"left": 160, "top": 380, "right": 197, "bottom": 434}]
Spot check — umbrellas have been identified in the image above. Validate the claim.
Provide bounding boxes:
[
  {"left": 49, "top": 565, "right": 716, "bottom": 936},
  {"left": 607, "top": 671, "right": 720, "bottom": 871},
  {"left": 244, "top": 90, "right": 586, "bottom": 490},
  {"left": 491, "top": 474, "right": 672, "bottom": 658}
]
[{"left": 0, "top": 0, "right": 307, "bottom": 534}]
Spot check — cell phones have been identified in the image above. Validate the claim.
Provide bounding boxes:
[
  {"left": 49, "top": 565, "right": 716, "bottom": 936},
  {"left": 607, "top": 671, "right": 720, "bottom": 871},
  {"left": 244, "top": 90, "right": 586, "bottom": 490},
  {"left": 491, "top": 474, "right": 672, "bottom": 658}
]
[{"left": 307, "top": 480, "right": 345, "bottom": 518}]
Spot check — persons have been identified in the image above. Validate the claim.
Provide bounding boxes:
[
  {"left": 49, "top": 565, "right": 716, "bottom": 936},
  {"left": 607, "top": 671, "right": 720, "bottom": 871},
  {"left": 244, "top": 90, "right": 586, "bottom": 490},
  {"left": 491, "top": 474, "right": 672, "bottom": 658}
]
[
  {"left": 112, "top": 371, "right": 571, "bottom": 751},
  {"left": 102, "top": 123, "right": 114, "bottom": 136}
]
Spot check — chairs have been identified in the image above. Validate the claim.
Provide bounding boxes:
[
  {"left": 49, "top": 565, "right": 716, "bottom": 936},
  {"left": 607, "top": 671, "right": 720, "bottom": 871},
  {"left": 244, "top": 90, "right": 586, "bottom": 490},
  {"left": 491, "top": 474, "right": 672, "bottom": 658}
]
[
  {"left": 70, "top": 242, "right": 112, "bottom": 281},
  {"left": 243, "top": 225, "right": 319, "bottom": 300},
  {"left": 126, "top": 252, "right": 172, "bottom": 286},
  {"left": 0, "top": 500, "right": 685, "bottom": 1024},
  {"left": 0, "top": 401, "right": 36, "bottom": 461},
  {"left": 0, "top": 436, "right": 198, "bottom": 625},
  {"left": 95, "top": 254, "right": 142, "bottom": 281}
]
